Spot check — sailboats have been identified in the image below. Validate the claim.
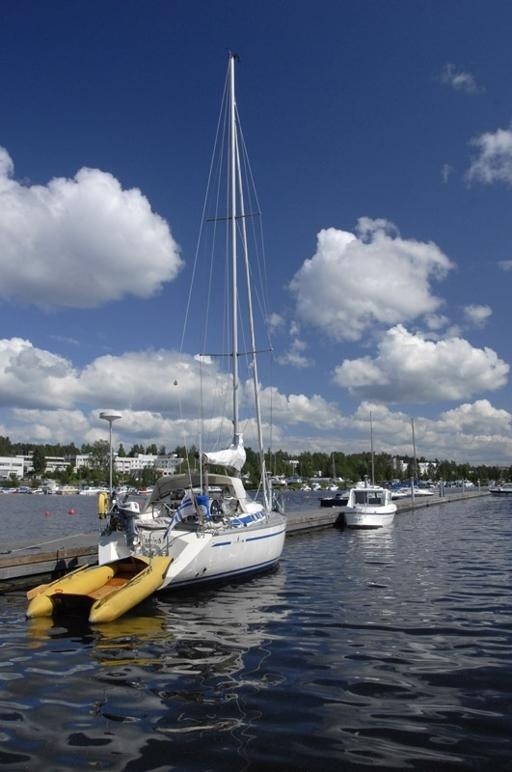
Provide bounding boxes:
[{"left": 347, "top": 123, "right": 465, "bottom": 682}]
[{"left": 98, "top": 50, "right": 288, "bottom": 590}]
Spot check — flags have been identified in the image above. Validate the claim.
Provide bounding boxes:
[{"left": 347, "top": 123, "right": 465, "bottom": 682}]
[{"left": 163, "top": 491, "right": 196, "bottom": 539}]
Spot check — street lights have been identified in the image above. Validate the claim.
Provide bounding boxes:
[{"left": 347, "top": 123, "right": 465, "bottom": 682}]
[{"left": 99, "top": 411, "right": 122, "bottom": 497}]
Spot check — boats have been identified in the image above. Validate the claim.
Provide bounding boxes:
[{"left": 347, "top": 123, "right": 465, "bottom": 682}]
[
  {"left": 25, "top": 555, "right": 173, "bottom": 623},
  {"left": 299, "top": 482, "right": 434, "bottom": 531},
  {"left": 489, "top": 485, "right": 512, "bottom": 493},
  {"left": 0, "top": 479, "right": 127, "bottom": 496}
]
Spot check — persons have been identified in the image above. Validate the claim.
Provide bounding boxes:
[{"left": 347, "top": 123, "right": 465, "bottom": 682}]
[{"left": 109, "top": 490, "right": 124, "bottom": 512}]
